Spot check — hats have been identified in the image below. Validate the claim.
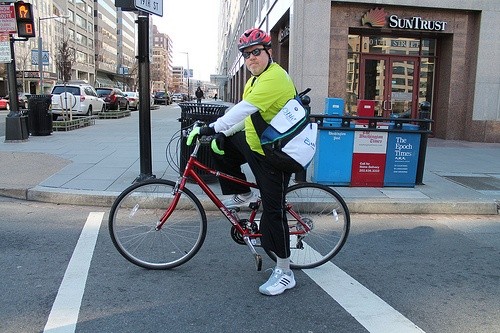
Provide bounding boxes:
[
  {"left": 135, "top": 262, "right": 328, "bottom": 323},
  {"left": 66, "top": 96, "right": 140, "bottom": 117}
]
[{"left": 198, "top": 86, "right": 200, "bottom": 89}]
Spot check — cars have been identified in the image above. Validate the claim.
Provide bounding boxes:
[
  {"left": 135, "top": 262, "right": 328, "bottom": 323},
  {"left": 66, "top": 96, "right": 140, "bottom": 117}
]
[
  {"left": 0, "top": 96, "right": 11, "bottom": 111},
  {"left": 7, "top": 92, "right": 30, "bottom": 109},
  {"left": 152, "top": 90, "right": 194, "bottom": 106},
  {"left": 122, "top": 91, "right": 155, "bottom": 110},
  {"left": 94, "top": 87, "right": 130, "bottom": 111}
]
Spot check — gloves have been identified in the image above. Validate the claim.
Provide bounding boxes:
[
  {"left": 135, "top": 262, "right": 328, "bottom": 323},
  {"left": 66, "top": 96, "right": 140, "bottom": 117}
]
[
  {"left": 210, "top": 133, "right": 227, "bottom": 155},
  {"left": 187, "top": 127, "right": 216, "bottom": 146}
]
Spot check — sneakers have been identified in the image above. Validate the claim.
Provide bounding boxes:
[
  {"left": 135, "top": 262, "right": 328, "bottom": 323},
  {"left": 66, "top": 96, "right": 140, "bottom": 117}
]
[
  {"left": 259, "top": 268, "right": 296, "bottom": 295},
  {"left": 221, "top": 190, "right": 258, "bottom": 208}
]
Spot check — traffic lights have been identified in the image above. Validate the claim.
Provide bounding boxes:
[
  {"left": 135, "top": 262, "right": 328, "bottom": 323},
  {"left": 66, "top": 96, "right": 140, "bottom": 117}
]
[{"left": 13, "top": 3, "right": 36, "bottom": 38}]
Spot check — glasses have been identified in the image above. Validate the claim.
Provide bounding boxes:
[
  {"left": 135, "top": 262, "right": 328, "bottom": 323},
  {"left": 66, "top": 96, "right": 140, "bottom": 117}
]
[{"left": 242, "top": 47, "right": 265, "bottom": 58}]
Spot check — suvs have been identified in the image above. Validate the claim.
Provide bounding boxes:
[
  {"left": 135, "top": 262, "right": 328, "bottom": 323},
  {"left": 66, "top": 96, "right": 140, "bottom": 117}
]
[{"left": 49, "top": 83, "right": 106, "bottom": 121}]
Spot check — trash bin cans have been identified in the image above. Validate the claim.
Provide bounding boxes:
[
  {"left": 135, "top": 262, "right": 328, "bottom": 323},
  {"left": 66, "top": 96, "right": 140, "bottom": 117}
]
[
  {"left": 25, "top": 95, "right": 53, "bottom": 136},
  {"left": 352, "top": 123, "right": 389, "bottom": 187},
  {"left": 177, "top": 102, "right": 230, "bottom": 183},
  {"left": 384, "top": 123, "right": 421, "bottom": 188},
  {"left": 309, "top": 121, "right": 357, "bottom": 187}
]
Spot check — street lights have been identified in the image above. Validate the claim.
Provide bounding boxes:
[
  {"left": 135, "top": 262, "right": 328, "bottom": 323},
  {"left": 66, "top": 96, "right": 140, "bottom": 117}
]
[
  {"left": 184, "top": 52, "right": 189, "bottom": 101},
  {"left": 37, "top": 16, "right": 70, "bottom": 95}
]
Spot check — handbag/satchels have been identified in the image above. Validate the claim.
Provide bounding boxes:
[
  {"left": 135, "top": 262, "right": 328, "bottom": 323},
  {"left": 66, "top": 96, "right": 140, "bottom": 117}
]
[{"left": 250, "top": 77, "right": 318, "bottom": 171}]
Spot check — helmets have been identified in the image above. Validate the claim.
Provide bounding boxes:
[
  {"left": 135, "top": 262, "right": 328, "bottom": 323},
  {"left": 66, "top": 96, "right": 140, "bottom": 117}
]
[{"left": 238, "top": 28, "right": 271, "bottom": 50}]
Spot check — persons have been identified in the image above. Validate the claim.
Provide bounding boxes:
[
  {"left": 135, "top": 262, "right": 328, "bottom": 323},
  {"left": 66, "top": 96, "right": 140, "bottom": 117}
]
[{"left": 186, "top": 29, "right": 296, "bottom": 296}]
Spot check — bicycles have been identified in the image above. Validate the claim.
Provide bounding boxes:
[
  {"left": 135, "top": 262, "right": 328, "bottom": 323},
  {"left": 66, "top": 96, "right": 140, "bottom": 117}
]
[{"left": 107, "top": 121, "right": 352, "bottom": 271}]
